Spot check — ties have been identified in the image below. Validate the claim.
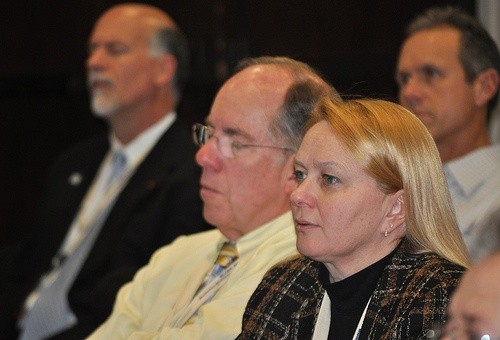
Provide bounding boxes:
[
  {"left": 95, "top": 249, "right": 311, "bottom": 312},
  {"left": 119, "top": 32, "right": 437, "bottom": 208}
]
[
  {"left": 194, "top": 241, "right": 238, "bottom": 306},
  {"left": 105, "top": 152, "right": 127, "bottom": 196}
]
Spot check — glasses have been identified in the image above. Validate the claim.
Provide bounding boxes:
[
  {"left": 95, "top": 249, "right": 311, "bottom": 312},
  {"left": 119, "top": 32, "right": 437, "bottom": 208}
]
[{"left": 191, "top": 123, "right": 294, "bottom": 157}]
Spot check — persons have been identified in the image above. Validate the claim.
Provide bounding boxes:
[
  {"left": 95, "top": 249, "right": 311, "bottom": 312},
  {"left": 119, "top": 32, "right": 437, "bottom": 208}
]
[
  {"left": 84, "top": 56, "right": 346, "bottom": 340},
  {"left": 396, "top": 8, "right": 500, "bottom": 256},
  {"left": 443, "top": 249, "right": 500, "bottom": 340},
  {"left": 235, "top": 100, "right": 472, "bottom": 340},
  {"left": 0, "top": 4, "right": 212, "bottom": 340}
]
